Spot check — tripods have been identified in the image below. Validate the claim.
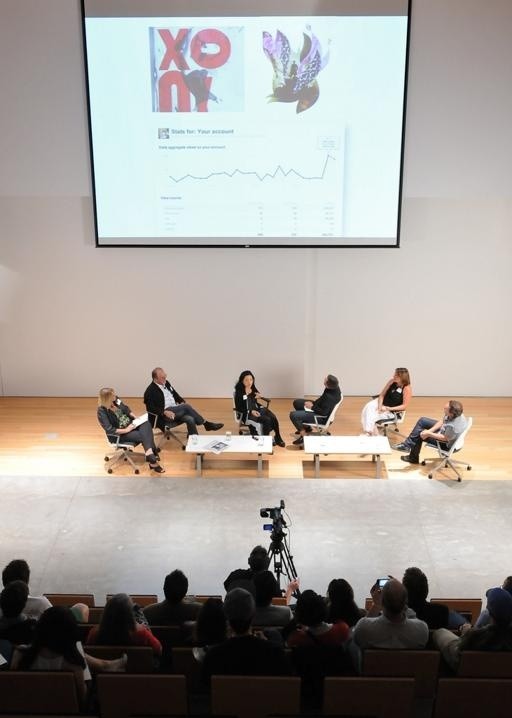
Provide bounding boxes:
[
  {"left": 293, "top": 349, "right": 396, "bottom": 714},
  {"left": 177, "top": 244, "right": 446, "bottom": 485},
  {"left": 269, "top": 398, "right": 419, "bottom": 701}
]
[{"left": 267, "top": 543, "right": 301, "bottom": 602}]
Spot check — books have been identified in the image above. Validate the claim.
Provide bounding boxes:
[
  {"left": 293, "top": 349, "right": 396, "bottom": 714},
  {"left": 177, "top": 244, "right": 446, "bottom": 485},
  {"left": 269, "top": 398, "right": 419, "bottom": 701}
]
[{"left": 131, "top": 412, "right": 149, "bottom": 429}]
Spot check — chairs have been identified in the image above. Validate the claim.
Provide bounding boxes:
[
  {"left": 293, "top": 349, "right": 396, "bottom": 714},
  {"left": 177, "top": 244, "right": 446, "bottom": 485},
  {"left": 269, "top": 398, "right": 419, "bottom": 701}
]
[
  {"left": 232, "top": 392, "right": 272, "bottom": 435},
  {"left": 422, "top": 417, "right": 472, "bottom": 482},
  {"left": 371, "top": 394, "right": 407, "bottom": 435},
  {"left": 104, "top": 429, "right": 142, "bottom": 474},
  {"left": 299, "top": 392, "right": 343, "bottom": 451},
  {"left": 146, "top": 411, "right": 189, "bottom": 452}
]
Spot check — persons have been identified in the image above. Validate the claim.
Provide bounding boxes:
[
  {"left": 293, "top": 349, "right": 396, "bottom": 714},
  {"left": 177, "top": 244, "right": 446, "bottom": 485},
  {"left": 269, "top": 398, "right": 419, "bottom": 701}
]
[
  {"left": 289, "top": 374, "right": 342, "bottom": 445},
  {"left": 391, "top": 399, "right": 467, "bottom": 465},
  {"left": 357, "top": 368, "right": 412, "bottom": 436},
  {"left": 96, "top": 386, "right": 167, "bottom": 474},
  {"left": 1, "top": 546, "right": 511, "bottom": 717},
  {"left": 233, "top": 370, "right": 286, "bottom": 447},
  {"left": 144, "top": 367, "right": 225, "bottom": 439}
]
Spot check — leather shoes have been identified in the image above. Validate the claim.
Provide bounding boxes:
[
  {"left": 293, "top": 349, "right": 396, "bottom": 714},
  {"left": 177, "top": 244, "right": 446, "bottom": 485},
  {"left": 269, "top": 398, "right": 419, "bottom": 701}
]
[
  {"left": 292, "top": 436, "right": 303, "bottom": 445},
  {"left": 203, "top": 421, "right": 224, "bottom": 432},
  {"left": 295, "top": 426, "right": 312, "bottom": 434},
  {"left": 273, "top": 436, "right": 286, "bottom": 447}
]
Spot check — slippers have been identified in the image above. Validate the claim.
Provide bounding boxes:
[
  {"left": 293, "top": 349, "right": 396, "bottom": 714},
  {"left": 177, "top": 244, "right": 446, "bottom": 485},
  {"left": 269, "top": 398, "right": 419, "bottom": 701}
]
[
  {"left": 145, "top": 454, "right": 156, "bottom": 464},
  {"left": 149, "top": 463, "right": 165, "bottom": 473}
]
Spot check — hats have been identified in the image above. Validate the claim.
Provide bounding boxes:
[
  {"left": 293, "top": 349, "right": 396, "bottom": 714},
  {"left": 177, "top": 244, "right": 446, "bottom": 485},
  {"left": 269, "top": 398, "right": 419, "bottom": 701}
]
[
  {"left": 222, "top": 579, "right": 257, "bottom": 624},
  {"left": 486, "top": 586, "right": 511, "bottom": 620}
]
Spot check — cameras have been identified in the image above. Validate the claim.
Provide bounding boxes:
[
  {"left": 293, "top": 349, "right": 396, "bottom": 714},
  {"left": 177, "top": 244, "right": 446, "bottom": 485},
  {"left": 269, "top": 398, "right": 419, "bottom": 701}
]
[{"left": 379, "top": 578, "right": 391, "bottom": 588}]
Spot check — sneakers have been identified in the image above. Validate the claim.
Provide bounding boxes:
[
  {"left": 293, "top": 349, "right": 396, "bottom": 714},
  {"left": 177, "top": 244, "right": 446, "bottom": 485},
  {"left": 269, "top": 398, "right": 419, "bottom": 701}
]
[
  {"left": 391, "top": 442, "right": 411, "bottom": 451},
  {"left": 116, "top": 653, "right": 133, "bottom": 674},
  {"left": 401, "top": 455, "right": 419, "bottom": 464}
]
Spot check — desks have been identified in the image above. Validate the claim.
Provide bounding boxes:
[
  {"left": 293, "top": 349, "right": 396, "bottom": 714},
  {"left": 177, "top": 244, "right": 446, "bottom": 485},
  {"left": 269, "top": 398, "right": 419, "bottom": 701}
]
[
  {"left": 303, "top": 435, "right": 392, "bottom": 479},
  {"left": 186, "top": 435, "right": 273, "bottom": 477}
]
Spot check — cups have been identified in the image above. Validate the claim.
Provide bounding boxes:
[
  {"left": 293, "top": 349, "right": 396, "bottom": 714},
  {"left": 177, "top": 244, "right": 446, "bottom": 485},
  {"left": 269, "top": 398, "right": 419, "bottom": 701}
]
[
  {"left": 258, "top": 437, "right": 264, "bottom": 446},
  {"left": 225, "top": 431, "right": 231, "bottom": 440},
  {"left": 192, "top": 435, "right": 198, "bottom": 445}
]
